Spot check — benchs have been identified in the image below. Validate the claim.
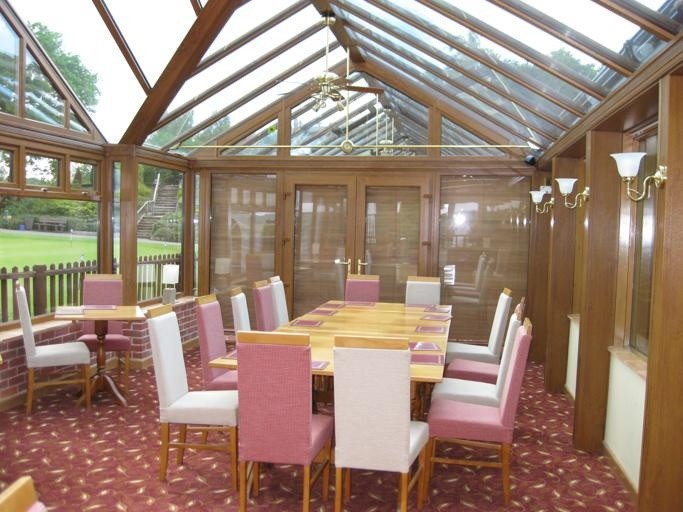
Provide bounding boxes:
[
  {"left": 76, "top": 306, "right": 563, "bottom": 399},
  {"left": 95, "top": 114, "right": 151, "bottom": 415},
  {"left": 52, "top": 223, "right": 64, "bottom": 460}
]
[{"left": 34, "top": 215, "right": 69, "bottom": 232}]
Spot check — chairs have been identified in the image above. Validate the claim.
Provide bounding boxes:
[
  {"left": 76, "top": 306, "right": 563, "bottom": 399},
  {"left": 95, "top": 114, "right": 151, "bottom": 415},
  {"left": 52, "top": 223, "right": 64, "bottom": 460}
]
[
  {"left": 14, "top": 280, "right": 93, "bottom": 415},
  {"left": 442, "top": 251, "right": 496, "bottom": 335},
  {"left": 77, "top": 275, "right": 131, "bottom": 395},
  {"left": 332, "top": 337, "right": 431, "bottom": 512},
  {"left": 405, "top": 275, "right": 441, "bottom": 305},
  {"left": 244, "top": 256, "right": 260, "bottom": 285},
  {"left": 230, "top": 275, "right": 290, "bottom": 349},
  {"left": 345, "top": 274, "right": 379, "bottom": 301},
  {"left": 235, "top": 330, "right": 334, "bottom": 512},
  {"left": 147, "top": 294, "right": 240, "bottom": 490},
  {"left": 424, "top": 288, "right": 533, "bottom": 506}
]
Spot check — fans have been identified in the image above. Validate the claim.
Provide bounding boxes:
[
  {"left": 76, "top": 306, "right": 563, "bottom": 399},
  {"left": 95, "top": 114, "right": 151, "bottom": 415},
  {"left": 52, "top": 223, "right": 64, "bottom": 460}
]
[
  {"left": 274, "top": 10, "right": 383, "bottom": 111},
  {"left": 369, "top": 107, "right": 416, "bottom": 156}
]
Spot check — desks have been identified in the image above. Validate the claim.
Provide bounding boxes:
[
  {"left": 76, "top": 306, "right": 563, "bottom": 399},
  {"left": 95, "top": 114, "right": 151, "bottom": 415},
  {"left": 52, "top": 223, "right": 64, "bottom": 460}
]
[
  {"left": 208, "top": 301, "right": 453, "bottom": 466},
  {"left": 54, "top": 305, "right": 145, "bottom": 411}
]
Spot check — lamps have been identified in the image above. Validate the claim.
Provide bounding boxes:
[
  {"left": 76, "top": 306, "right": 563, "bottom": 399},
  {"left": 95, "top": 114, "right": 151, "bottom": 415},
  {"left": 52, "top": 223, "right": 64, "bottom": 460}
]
[
  {"left": 555, "top": 178, "right": 590, "bottom": 209},
  {"left": 161, "top": 264, "right": 179, "bottom": 306},
  {"left": 213, "top": 258, "right": 230, "bottom": 291},
  {"left": 529, "top": 191, "right": 554, "bottom": 215},
  {"left": 610, "top": 152, "right": 667, "bottom": 202}
]
